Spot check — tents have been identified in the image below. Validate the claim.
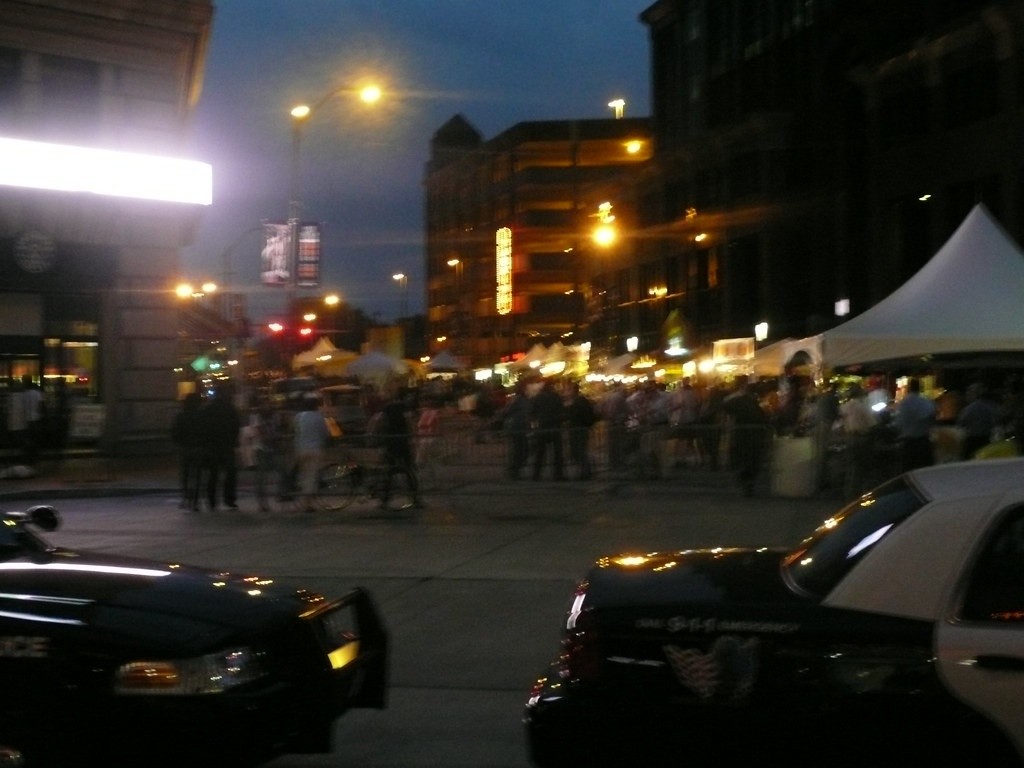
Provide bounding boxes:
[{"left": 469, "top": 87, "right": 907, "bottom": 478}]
[{"left": 296, "top": 206, "right": 1024, "bottom": 387}]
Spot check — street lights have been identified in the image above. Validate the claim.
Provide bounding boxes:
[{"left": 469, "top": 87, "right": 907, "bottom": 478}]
[{"left": 283, "top": 74, "right": 386, "bottom": 377}]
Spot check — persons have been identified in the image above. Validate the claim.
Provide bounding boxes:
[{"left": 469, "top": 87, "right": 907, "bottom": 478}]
[{"left": 6, "top": 371, "right": 1001, "bottom": 514}]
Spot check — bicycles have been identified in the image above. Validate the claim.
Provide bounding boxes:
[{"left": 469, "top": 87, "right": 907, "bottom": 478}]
[{"left": 311, "top": 432, "right": 424, "bottom": 514}]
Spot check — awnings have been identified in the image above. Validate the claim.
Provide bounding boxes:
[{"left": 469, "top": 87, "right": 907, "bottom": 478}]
[{"left": 0, "top": 290, "right": 48, "bottom": 336}]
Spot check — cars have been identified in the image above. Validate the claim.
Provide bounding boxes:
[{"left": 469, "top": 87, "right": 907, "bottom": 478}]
[
  {"left": 520, "top": 451, "right": 1023, "bottom": 768},
  {"left": 0, "top": 502, "right": 364, "bottom": 768},
  {"left": 266, "top": 374, "right": 326, "bottom": 415}
]
